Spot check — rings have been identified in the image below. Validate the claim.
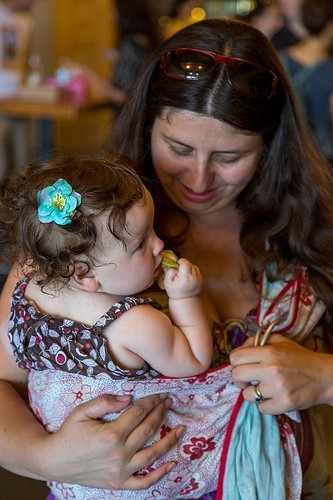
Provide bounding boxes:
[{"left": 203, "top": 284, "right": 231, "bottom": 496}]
[{"left": 254, "top": 384, "right": 263, "bottom": 399}]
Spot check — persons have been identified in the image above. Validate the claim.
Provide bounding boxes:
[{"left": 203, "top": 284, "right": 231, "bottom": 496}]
[
  {"left": 1, "top": 0, "right": 35, "bottom": 138},
  {"left": 1, "top": 18, "right": 333, "bottom": 491},
  {"left": 1, "top": 158, "right": 214, "bottom": 500},
  {"left": 74, "top": 0, "right": 333, "bottom": 162}
]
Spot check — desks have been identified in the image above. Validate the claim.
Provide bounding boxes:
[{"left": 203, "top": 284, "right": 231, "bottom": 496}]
[{"left": 0, "top": 101, "right": 117, "bottom": 159}]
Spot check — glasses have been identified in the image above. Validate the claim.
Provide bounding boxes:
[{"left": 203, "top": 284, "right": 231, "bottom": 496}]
[{"left": 158, "top": 46, "right": 280, "bottom": 100}]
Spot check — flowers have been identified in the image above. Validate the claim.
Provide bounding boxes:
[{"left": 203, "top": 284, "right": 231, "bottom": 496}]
[{"left": 36, "top": 177, "right": 82, "bottom": 227}]
